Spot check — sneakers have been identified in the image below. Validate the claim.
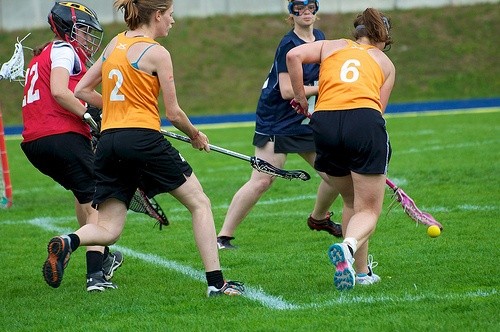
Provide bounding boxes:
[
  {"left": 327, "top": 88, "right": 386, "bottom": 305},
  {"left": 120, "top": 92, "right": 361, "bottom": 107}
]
[
  {"left": 86, "top": 271, "right": 118, "bottom": 292},
  {"left": 103, "top": 250, "right": 126, "bottom": 277},
  {"left": 328, "top": 242, "right": 356, "bottom": 290},
  {"left": 205, "top": 279, "right": 244, "bottom": 296},
  {"left": 217, "top": 235, "right": 236, "bottom": 250},
  {"left": 355, "top": 255, "right": 381, "bottom": 286},
  {"left": 307, "top": 211, "right": 343, "bottom": 237},
  {"left": 42, "top": 234, "right": 72, "bottom": 289}
]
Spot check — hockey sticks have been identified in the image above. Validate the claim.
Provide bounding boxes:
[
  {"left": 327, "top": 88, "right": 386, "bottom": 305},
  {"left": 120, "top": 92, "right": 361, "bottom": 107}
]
[
  {"left": 161, "top": 129, "right": 310, "bottom": 183},
  {"left": 385, "top": 174, "right": 445, "bottom": 231},
  {"left": 84, "top": 114, "right": 169, "bottom": 229}
]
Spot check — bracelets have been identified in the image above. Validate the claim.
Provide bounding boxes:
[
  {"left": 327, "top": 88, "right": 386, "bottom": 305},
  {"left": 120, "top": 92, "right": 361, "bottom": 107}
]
[{"left": 189, "top": 130, "right": 205, "bottom": 140}]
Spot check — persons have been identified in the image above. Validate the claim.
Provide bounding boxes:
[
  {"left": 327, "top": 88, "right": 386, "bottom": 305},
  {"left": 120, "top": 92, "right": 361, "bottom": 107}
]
[
  {"left": 41, "top": 0, "right": 246, "bottom": 298},
  {"left": 21, "top": 0, "right": 125, "bottom": 292},
  {"left": 285, "top": 6, "right": 397, "bottom": 290},
  {"left": 216, "top": 0, "right": 343, "bottom": 251}
]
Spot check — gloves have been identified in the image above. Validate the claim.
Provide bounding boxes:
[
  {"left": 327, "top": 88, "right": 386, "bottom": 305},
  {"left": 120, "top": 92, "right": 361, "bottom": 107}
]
[{"left": 83, "top": 107, "right": 103, "bottom": 137}]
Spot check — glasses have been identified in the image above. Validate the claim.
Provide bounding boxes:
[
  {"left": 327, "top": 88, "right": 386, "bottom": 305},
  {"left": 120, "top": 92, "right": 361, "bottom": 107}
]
[
  {"left": 288, "top": 0, "right": 320, "bottom": 15},
  {"left": 383, "top": 42, "right": 391, "bottom": 51}
]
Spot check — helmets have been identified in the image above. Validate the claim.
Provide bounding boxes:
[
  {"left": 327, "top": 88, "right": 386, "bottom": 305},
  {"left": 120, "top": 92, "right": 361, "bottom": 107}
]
[{"left": 47, "top": 0, "right": 104, "bottom": 65}]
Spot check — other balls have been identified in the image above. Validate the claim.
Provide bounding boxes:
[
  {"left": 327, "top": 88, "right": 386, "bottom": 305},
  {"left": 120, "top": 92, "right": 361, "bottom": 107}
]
[{"left": 427, "top": 226, "right": 441, "bottom": 237}]
